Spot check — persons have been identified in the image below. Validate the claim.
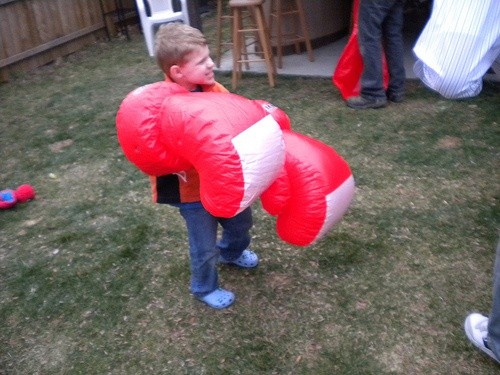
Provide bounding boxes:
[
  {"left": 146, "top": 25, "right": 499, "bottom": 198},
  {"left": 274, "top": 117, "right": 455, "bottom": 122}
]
[
  {"left": 464, "top": 232, "right": 500, "bottom": 363},
  {"left": 345, "top": 0, "right": 406, "bottom": 108},
  {"left": 153, "top": 22, "right": 258, "bottom": 308}
]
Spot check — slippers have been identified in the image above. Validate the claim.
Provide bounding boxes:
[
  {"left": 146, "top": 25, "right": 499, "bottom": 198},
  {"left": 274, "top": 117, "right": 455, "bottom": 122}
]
[
  {"left": 217, "top": 249, "right": 258, "bottom": 268},
  {"left": 194, "top": 287, "right": 235, "bottom": 309}
]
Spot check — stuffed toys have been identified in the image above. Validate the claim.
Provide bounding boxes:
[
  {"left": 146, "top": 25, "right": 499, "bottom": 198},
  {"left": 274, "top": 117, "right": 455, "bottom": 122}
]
[{"left": 0, "top": 184, "right": 41, "bottom": 208}]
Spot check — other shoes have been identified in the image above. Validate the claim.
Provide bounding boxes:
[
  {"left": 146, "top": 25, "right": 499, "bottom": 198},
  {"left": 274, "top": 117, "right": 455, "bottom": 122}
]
[
  {"left": 346, "top": 94, "right": 387, "bottom": 109},
  {"left": 385, "top": 91, "right": 403, "bottom": 102}
]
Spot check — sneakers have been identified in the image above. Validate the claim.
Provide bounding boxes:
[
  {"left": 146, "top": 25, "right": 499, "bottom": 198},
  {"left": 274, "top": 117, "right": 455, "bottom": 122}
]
[{"left": 464, "top": 312, "right": 500, "bottom": 362}]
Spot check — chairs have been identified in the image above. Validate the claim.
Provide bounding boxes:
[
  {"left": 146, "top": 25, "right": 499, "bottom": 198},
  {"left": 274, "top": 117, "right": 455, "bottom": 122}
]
[{"left": 136, "top": 0, "right": 190, "bottom": 56}]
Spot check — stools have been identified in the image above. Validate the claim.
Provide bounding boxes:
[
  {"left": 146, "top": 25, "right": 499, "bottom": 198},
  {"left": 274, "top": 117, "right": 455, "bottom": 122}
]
[
  {"left": 100, "top": 0, "right": 143, "bottom": 42},
  {"left": 215, "top": 0, "right": 315, "bottom": 89}
]
